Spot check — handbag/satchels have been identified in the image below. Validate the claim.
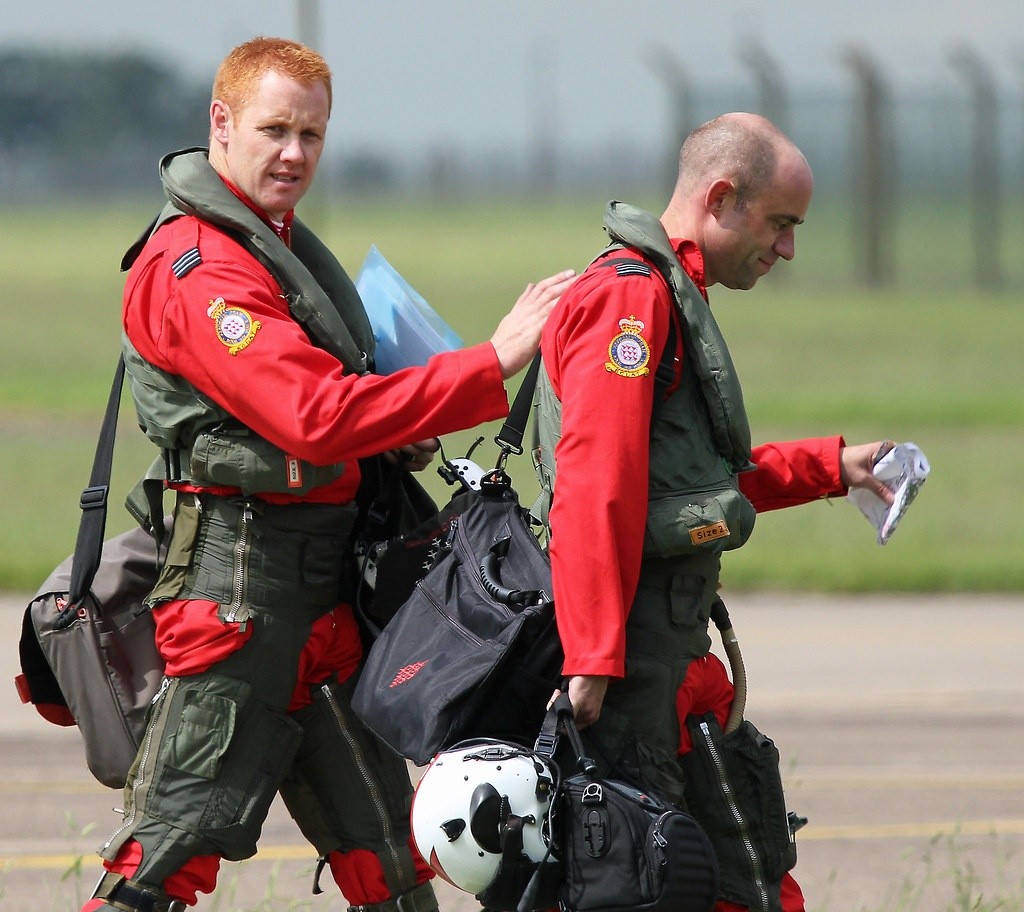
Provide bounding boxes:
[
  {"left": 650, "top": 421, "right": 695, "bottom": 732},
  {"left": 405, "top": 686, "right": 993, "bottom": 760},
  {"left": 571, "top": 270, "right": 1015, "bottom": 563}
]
[
  {"left": 16, "top": 349, "right": 177, "bottom": 788},
  {"left": 351, "top": 348, "right": 565, "bottom": 767},
  {"left": 677, "top": 720, "right": 807, "bottom": 911},
  {"left": 554, "top": 694, "right": 724, "bottom": 911}
]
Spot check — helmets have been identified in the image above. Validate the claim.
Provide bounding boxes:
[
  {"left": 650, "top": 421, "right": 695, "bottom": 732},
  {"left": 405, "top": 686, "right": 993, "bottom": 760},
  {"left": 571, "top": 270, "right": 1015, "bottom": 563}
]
[{"left": 410, "top": 737, "right": 560, "bottom": 911}]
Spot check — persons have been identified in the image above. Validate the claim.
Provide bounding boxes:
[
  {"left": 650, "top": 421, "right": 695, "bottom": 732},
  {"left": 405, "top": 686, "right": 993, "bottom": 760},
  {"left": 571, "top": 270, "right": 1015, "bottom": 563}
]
[
  {"left": 532, "top": 112, "right": 901, "bottom": 912},
  {"left": 80, "top": 39, "right": 583, "bottom": 912}
]
